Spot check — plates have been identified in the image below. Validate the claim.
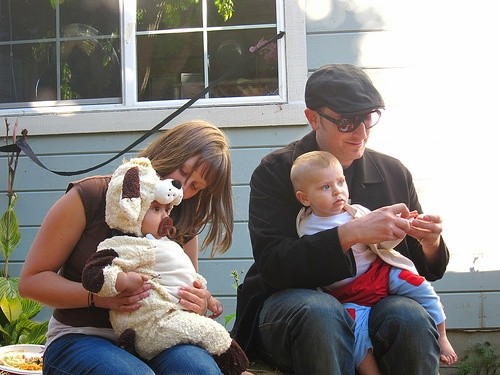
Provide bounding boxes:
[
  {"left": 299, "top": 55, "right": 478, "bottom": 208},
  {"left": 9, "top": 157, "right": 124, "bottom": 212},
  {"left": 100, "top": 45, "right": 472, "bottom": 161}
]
[{"left": 0, "top": 343, "right": 46, "bottom": 375}]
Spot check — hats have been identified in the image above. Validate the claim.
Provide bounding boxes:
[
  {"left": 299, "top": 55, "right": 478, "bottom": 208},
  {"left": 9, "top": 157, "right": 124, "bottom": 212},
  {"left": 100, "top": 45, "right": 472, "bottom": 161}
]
[{"left": 304, "top": 63, "right": 386, "bottom": 114}]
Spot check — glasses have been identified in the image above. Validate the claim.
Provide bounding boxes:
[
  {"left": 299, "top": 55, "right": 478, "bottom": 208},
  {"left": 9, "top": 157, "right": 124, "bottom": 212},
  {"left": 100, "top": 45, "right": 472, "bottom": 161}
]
[{"left": 314, "top": 109, "right": 381, "bottom": 134}]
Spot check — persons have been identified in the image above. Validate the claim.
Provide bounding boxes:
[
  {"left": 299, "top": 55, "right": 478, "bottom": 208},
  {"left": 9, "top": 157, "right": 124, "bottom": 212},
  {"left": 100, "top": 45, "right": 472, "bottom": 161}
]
[
  {"left": 17, "top": 118, "right": 235, "bottom": 375},
  {"left": 81, "top": 156, "right": 255, "bottom": 375},
  {"left": 236, "top": 63, "right": 450, "bottom": 374},
  {"left": 289, "top": 150, "right": 458, "bottom": 375}
]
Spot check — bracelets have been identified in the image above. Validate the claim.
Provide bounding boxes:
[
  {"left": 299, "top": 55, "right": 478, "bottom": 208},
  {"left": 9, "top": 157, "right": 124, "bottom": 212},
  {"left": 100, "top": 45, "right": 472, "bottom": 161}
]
[{"left": 87, "top": 290, "right": 96, "bottom": 309}]
